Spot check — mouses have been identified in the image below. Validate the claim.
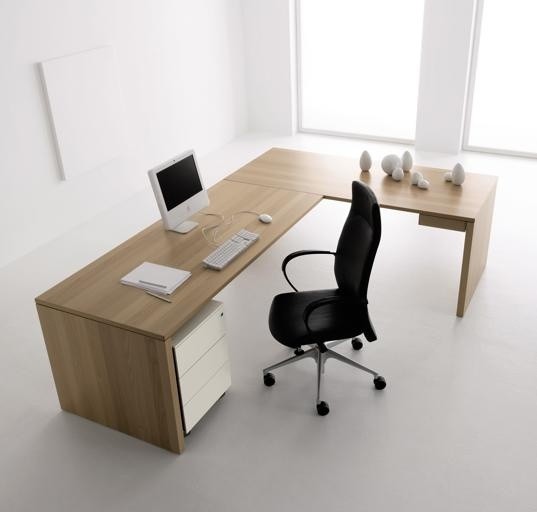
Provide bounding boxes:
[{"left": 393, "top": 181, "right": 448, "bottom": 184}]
[{"left": 258, "top": 214, "right": 272, "bottom": 224}]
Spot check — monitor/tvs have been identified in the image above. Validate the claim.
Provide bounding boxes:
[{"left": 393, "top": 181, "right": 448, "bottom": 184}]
[{"left": 148, "top": 150, "right": 210, "bottom": 234}]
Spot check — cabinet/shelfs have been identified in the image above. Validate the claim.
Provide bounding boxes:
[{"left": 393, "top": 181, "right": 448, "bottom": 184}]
[{"left": 164, "top": 299, "right": 233, "bottom": 436}]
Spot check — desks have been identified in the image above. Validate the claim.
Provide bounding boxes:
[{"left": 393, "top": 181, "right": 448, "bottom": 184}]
[{"left": 33, "top": 145, "right": 500, "bottom": 456}]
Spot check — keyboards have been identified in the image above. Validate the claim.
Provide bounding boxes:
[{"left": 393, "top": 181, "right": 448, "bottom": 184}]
[{"left": 202, "top": 228, "right": 259, "bottom": 270}]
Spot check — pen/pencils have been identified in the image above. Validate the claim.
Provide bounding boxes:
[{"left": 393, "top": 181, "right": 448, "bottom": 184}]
[
  {"left": 145, "top": 291, "right": 172, "bottom": 303},
  {"left": 139, "top": 280, "right": 167, "bottom": 288}
]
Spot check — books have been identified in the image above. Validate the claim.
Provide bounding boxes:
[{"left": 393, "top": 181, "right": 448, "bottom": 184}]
[{"left": 120, "top": 262, "right": 192, "bottom": 296}]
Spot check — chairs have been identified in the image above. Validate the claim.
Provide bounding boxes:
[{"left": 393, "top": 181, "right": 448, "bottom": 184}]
[{"left": 263, "top": 179, "right": 386, "bottom": 415}]
